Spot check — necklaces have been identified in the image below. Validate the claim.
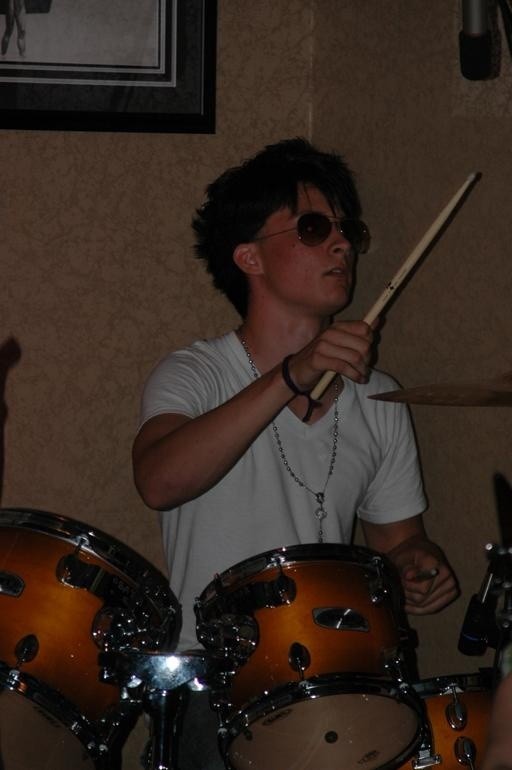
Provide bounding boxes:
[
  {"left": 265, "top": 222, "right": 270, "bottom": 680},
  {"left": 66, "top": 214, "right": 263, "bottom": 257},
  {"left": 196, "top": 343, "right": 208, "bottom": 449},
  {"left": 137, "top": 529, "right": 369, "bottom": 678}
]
[{"left": 235, "top": 323, "right": 339, "bottom": 546}]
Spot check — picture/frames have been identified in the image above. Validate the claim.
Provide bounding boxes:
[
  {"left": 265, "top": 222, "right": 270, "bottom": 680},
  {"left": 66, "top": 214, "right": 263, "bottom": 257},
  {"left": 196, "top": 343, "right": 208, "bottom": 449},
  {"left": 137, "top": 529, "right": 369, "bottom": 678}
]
[{"left": 0, "top": 0, "right": 218, "bottom": 134}]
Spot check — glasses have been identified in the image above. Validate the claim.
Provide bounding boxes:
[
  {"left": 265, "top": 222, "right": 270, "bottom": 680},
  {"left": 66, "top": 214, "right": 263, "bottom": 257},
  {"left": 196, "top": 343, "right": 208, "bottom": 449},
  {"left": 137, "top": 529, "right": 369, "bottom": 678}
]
[{"left": 242, "top": 209, "right": 371, "bottom": 254}]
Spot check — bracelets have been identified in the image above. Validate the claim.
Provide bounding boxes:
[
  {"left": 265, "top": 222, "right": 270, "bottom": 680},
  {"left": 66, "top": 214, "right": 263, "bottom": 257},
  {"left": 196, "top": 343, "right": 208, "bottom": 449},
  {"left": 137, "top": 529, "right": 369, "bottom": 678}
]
[{"left": 283, "top": 352, "right": 310, "bottom": 396}]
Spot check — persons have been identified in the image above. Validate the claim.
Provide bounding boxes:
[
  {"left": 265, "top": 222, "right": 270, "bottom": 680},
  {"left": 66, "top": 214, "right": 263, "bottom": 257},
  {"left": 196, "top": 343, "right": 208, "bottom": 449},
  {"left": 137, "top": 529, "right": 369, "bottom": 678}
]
[{"left": 131, "top": 135, "right": 463, "bottom": 766}]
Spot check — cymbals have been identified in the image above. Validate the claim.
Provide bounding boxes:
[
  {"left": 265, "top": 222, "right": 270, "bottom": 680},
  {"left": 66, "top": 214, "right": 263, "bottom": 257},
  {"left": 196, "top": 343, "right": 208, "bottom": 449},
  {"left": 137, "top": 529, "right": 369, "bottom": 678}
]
[{"left": 366, "top": 384, "right": 510, "bottom": 407}]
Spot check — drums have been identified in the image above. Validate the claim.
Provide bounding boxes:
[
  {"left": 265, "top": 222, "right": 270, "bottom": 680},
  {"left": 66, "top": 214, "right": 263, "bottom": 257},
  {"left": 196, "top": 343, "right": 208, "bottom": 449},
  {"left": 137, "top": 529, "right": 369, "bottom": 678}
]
[
  {"left": 1, "top": 507, "right": 181, "bottom": 769},
  {"left": 192, "top": 542, "right": 426, "bottom": 770},
  {"left": 399, "top": 675, "right": 501, "bottom": 770}
]
